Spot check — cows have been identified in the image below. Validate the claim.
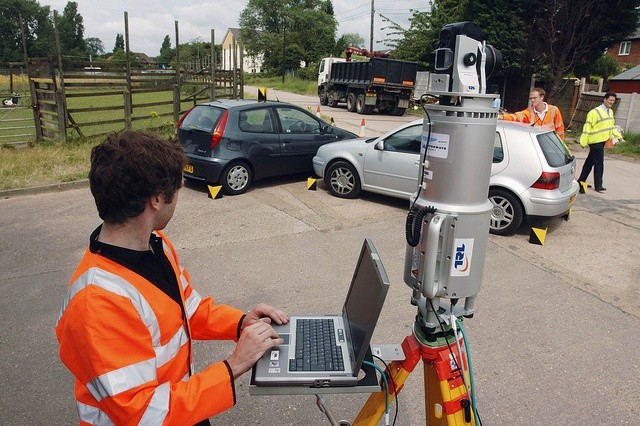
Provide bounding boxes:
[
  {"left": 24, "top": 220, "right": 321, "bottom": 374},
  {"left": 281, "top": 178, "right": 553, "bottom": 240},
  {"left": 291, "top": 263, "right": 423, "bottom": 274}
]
[{"left": 2, "top": 92, "right": 21, "bottom": 107}]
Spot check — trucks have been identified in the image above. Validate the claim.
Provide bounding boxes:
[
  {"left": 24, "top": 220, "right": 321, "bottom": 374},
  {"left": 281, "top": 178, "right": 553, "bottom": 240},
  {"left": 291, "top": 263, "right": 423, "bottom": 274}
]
[{"left": 317, "top": 48, "right": 418, "bottom": 116}]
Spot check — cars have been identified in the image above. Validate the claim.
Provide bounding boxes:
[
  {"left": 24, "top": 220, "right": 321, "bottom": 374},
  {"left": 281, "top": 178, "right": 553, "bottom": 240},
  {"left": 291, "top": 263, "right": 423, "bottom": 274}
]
[
  {"left": 312, "top": 119, "right": 580, "bottom": 235},
  {"left": 176, "top": 100, "right": 360, "bottom": 195}
]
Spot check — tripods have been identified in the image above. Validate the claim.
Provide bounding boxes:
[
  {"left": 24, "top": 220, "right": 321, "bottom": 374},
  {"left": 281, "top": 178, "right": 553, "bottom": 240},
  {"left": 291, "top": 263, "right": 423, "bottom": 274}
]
[{"left": 352, "top": 319, "right": 476, "bottom": 426}]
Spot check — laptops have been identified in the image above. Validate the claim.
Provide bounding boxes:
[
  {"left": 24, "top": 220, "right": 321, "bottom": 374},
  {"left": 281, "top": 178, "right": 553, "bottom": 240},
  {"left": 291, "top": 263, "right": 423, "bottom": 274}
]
[{"left": 255, "top": 238, "right": 390, "bottom": 386}]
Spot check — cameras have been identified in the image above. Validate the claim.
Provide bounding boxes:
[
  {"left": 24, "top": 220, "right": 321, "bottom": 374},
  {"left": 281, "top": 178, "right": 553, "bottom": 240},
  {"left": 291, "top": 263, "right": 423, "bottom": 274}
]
[{"left": 431, "top": 21, "right": 503, "bottom": 106}]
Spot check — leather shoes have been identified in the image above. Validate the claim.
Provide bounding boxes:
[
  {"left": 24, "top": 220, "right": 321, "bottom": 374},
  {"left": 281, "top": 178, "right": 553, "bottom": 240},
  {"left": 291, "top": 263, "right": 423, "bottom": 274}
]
[
  {"left": 587, "top": 185, "right": 591, "bottom": 188},
  {"left": 595, "top": 186, "right": 606, "bottom": 192}
]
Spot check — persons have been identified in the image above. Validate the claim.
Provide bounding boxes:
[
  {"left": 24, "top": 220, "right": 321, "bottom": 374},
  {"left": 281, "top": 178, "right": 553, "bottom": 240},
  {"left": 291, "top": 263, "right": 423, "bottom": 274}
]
[
  {"left": 577, "top": 93, "right": 622, "bottom": 191},
  {"left": 492, "top": 91, "right": 502, "bottom": 115},
  {"left": 53, "top": 130, "right": 289, "bottom": 426},
  {"left": 498, "top": 88, "right": 565, "bottom": 141}
]
